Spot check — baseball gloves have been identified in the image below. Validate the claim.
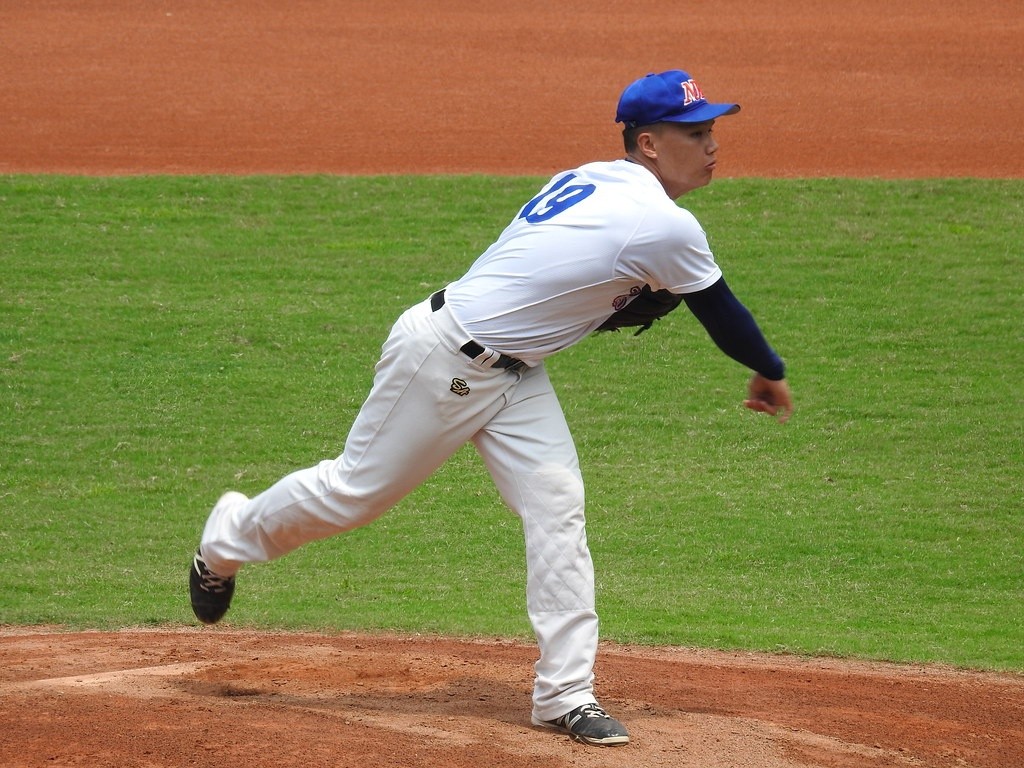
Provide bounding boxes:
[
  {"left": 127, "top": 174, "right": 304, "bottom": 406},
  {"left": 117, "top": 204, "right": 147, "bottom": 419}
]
[{"left": 592, "top": 283, "right": 684, "bottom": 339}]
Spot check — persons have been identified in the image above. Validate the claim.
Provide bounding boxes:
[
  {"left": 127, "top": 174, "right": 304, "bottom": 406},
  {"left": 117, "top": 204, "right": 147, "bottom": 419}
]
[{"left": 189, "top": 71, "right": 794, "bottom": 746}]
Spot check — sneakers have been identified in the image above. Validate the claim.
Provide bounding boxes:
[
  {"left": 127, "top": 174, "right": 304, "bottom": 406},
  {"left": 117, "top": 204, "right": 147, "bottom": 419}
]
[
  {"left": 190, "top": 547, "right": 235, "bottom": 625},
  {"left": 531, "top": 702, "right": 629, "bottom": 745}
]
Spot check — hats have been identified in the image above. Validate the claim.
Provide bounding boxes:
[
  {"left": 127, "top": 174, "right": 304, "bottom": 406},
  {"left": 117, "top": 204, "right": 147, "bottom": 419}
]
[{"left": 616, "top": 70, "right": 741, "bottom": 131}]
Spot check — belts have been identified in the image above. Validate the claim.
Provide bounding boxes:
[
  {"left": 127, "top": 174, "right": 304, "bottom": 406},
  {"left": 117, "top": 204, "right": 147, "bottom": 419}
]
[{"left": 430, "top": 291, "right": 524, "bottom": 372}]
